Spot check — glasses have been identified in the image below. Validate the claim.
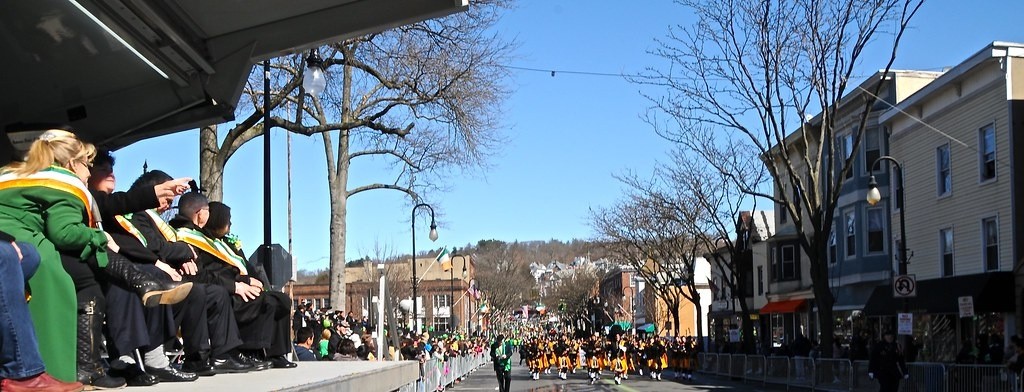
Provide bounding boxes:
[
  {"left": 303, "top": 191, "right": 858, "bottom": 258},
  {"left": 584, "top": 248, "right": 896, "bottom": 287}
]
[{"left": 77, "top": 158, "right": 94, "bottom": 169}]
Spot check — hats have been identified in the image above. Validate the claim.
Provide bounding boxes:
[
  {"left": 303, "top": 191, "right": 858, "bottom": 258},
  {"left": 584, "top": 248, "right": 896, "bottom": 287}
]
[{"left": 337, "top": 320, "right": 350, "bottom": 328}]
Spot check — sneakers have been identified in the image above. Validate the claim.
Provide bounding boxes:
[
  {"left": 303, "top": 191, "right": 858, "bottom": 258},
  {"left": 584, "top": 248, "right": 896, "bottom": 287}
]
[{"left": 227, "top": 353, "right": 269, "bottom": 370}]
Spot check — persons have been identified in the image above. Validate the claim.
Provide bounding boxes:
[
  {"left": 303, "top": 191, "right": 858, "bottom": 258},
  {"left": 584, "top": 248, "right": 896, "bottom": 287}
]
[
  {"left": 1, "top": 127, "right": 193, "bottom": 390},
  {"left": 711, "top": 329, "right": 1023, "bottom": 392},
  {"left": 294, "top": 292, "right": 709, "bottom": 392},
  {"left": 0, "top": 229, "right": 86, "bottom": 392},
  {"left": 81, "top": 146, "right": 298, "bottom": 387}
]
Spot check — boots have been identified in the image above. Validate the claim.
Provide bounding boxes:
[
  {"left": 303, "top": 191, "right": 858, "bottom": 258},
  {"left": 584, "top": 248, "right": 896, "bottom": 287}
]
[
  {"left": 588, "top": 370, "right": 601, "bottom": 384},
  {"left": 104, "top": 251, "right": 194, "bottom": 308},
  {"left": 74, "top": 298, "right": 127, "bottom": 391},
  {"left": 638, "top": 367, "right": 646, "bottom": 377},
  {"left": 544, "top": 368, "right": 552, "bottom": 375},
  {"left": 532, "top": 373, "right": 540, "bottom": 380},
  {"left": 560, "top": 372, "right": 567, "bottom": 380},
  {"left": 650, "top": 371, "right": 662, "bottom": 381},
  {"left": 621, "top": 374, "right": 629, "bottom": 380},
  {"left": 675, "top": 370, "right": 692, "bottom": 381},
  {"left": 615, "top": 376, "right": 622, "bottom": 385}
]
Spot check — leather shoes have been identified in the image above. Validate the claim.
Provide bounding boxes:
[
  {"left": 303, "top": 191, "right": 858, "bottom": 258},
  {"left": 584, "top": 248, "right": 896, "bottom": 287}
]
[
  {"left": 144, "top": 362, "right": 198, "bottom": 382},
  {"left": 2, "top": 372, "right": 84, "bottom": 392},
  {"left": 112, "top": 361, "right": 159, "bottom": 386},
  {"left": 183, "top": 363, "right": 217, "bottom": 376},
  {"left": 214, "top": 359, "right": 252, "bottom": 373},
  {"left": 266, "top": 356, "right": 297, "bottom": 368}
]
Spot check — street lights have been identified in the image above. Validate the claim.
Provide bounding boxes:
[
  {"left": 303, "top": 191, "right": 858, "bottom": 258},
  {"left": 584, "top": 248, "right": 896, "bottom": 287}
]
[
  {"left": 476, "top": 290, "right": 488, "bottom": 335},
  {"left": 450, "top": 254, "right": 468, "bottom": 331},
  {"left": 621, "top": 285, "right": 636, "bottom": 329},
  {"left": 262, "top": 46, "right": 326, "bottom": 286},
  {"left": 866, "top": 155, "right": 915, "bottom": 362},
  {"left": 469, "top": 277, "right": 481, "bottom": 336},
  {"left": 412, "top": 203, "right": 438, "bottom": 331}
]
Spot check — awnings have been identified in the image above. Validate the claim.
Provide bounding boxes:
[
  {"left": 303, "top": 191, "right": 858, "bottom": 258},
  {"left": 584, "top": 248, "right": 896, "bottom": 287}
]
[{"left": 758, "top": 298, "right": 808, "bottom": 314}]
